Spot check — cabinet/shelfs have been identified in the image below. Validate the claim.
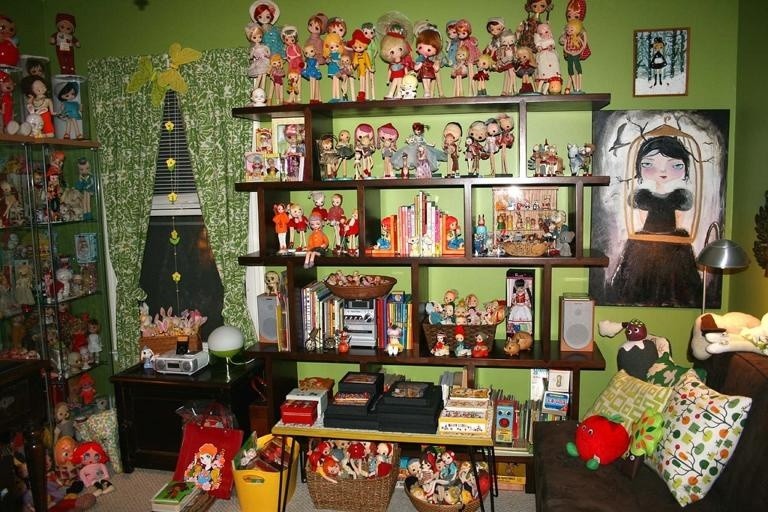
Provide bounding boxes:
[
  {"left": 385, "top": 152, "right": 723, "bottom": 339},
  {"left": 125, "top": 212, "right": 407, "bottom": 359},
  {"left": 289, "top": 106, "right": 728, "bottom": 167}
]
[
  {"left": 0, "top": 133, "right": 57, "bottom": 456},
  {"left": 35, "top": 132, "right": 123, "bottom": 433},
  {"left": 231, "top": 91, "right": 617, "bottom": 497},
  {"left": 110, "top": 352, "right": 265, "bottom": 475}
]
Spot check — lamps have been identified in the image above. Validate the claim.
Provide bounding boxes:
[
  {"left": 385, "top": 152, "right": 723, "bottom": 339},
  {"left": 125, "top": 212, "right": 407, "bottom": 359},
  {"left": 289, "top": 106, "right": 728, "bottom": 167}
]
[{"left": 696, "top": 220, "right": 754, "bottom": 319}]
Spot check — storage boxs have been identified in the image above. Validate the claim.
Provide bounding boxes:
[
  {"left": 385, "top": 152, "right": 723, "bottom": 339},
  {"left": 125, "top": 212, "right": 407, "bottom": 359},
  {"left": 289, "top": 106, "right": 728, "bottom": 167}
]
[{"left": 231, "top": 432, "right": 301, "bottom": 511}]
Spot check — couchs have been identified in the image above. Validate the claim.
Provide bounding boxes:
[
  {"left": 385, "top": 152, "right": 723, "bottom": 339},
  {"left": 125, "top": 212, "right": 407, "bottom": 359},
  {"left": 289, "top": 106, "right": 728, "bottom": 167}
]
[{"left": 529, "top": 342, "right": 767, "bottom": 511}]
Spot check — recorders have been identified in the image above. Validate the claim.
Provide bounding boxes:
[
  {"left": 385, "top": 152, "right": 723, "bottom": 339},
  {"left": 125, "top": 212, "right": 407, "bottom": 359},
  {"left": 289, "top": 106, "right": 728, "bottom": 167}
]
[{"left": 149, "top": 351, "right": 208, "bottom": 376}]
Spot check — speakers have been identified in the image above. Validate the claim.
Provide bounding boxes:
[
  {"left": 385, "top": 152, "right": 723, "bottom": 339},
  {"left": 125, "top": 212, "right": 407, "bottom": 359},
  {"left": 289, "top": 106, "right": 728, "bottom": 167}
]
[
  {"left": 257, "top": 294, "right": 279, "bottom": 343},
  {"left": 559, "top": 295, "right": 595, "bottom": 352}
]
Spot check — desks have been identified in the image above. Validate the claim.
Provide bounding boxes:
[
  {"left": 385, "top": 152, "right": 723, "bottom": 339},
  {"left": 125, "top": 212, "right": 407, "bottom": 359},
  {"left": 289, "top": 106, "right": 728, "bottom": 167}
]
[
  {"left": 271, "top": 415, "right": 500, "bottom": 512},
  {"left": 0, "top": 356, "right": 57, "bottom": 510}
]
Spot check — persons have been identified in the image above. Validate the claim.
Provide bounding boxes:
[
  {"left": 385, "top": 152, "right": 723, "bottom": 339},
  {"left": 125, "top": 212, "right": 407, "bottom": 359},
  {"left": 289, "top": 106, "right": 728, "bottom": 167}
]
[
  {"left": 0, "top": 11, "right": 114, "bottom": 512},
  {"left": 373, "top": 217, "right": 392, "bottom": 249},
  {"left": 242, "top": 1, "right": 591, "bottom": 106},
  {"left": 445, "top": 216, "right": 465, "bottom": 249},
  {"left": 242, "top": 0, "right": 596, "bottom": 506},
  {"left": 306, "top": 438, "right": 395, "bottom": 484},
  {"left": 406, "top": 447, "right": 491, "bottom": 506},
  {"left": 3, "top": 12, "right": 113, "bottom": 512},
  {"left": 273, "top": 191, "right": 360, "bottom": 269},
  {"left": 425, "top": 278, "right": 533, "bottom": 357},
  {"left": 242, "top": 115, "right": 513, "bottom": 182},
  {"left": 649, "top": 35, "right": 668, "bottom": 86},
  {"left": 608, "top": 135, "right": 712, "bottom": 304}
]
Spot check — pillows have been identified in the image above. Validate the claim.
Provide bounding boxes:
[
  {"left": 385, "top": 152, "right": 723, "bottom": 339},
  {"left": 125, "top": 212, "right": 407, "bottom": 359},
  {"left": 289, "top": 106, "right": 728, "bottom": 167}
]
[
  {"left": 640, "top": 368, "right": 754, "bottom": 510},
  {"left": 582, "top": 368, "right": 673, "bottom": 463},
  {"left": 644, "top": 353, "right": 708, "bottom": 391}
]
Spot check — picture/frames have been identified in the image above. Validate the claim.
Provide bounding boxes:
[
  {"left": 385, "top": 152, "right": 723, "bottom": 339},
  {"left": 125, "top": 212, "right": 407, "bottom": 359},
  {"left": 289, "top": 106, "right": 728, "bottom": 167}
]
[{"left": 631, "top": 27, "right": 691, "bottom": 99}]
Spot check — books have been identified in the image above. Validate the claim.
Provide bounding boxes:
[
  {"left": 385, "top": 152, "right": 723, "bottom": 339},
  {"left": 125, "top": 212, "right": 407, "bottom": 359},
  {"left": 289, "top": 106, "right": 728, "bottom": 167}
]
[
  {"left": 300, "top": 280, "right": 343, "bottom": 351},
  {"left": 150, "top": 480, "right": 216, "bottom": 512},
  {"left": 377, "top": 293, "right": 413, "bottom": 351}
]
